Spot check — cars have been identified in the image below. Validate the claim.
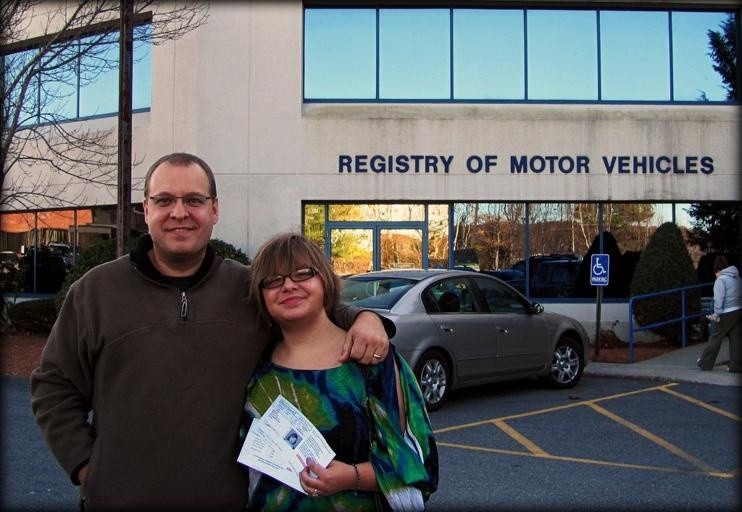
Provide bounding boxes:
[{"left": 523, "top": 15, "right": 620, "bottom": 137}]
[
  {"left": 451, "top": 255, "right": 584, "bottom": 299},
  {"left": 342, "top": 269, "right": 588, "bottom": 410}
]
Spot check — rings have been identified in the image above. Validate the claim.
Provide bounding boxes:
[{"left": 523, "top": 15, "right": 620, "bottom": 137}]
[
  {"left": 372, "top": 353, "right": 383, "bottom": 360},
  {"left": 312, "top": 488, "right": 319, "bottom": 498}
]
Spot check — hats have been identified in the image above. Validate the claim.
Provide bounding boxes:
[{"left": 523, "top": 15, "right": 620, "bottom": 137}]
[{"left": 714, "top": 256, "right": 728, "bottom": 272}]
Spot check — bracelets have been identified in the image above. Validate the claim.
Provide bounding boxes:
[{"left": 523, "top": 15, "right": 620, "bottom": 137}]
[{"left": 350, "top": 462, "right": 361, "bottom": 493}]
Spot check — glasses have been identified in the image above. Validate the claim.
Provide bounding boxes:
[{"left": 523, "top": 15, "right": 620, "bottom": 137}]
[
  {"left": 259, "top": 267, "right": 318, "bottom": 289},
  {"left": 149, "top": 193, "right": 215, "bottom": 207}
]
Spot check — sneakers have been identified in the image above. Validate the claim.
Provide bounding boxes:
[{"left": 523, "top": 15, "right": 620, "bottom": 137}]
[{"left": 697, "top": 357, "right": 742, "bottom": 372}]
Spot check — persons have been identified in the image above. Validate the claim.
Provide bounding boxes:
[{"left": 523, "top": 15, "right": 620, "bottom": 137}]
[
  {"left": 232, "top": 233, "right": 439, "bottom": 511},
  {"left": 695, "top": 254, "right": 742, "bottom": 373},
  {"left": 28, "top": 153, "right": 396, "bottom": 511},
  {"left": 283, "top": 433, "right": 297, "bottom": 449}
]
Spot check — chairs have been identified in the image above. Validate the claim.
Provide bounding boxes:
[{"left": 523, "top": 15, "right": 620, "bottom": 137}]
[{"left": 435, "top": 292, "right": 461, "bottom": 314}]
